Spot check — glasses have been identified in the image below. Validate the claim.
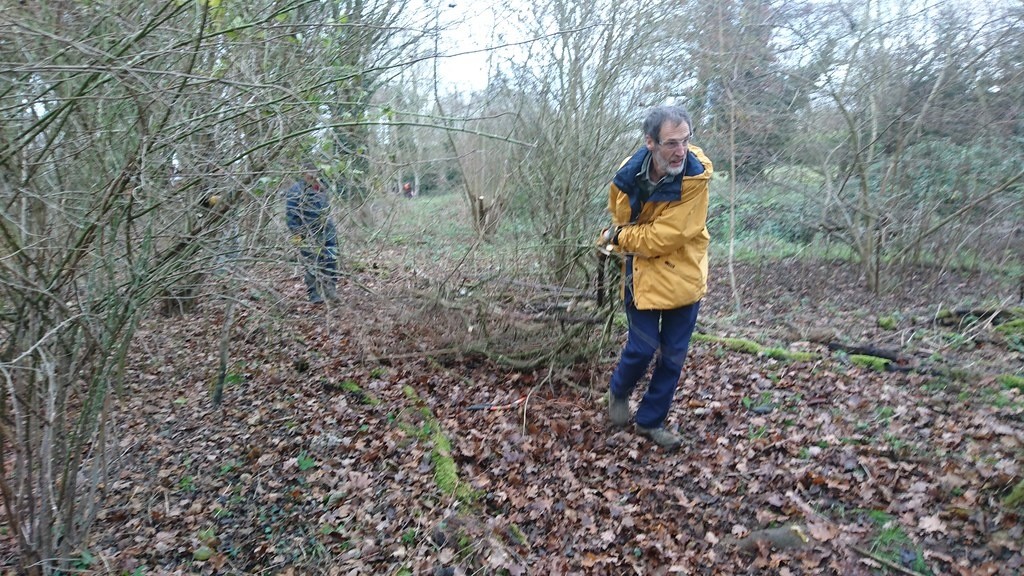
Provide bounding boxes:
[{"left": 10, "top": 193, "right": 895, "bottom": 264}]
[{"left": 653, "top": 133, "right": 694, "bottom": 148}]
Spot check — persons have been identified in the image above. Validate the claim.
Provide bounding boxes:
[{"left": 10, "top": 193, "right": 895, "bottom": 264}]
[
  {"left": 405, "top": 182, "right": 412, "bottom": 198},
  {"left": 285, "top": 163, "right": 341, "bottom": 305},
  {"left": 156, "top": 135, "right": 222, "bottom": 316},
  {"left": 598, "top": 106, "right": 713, "bottom": 451}
]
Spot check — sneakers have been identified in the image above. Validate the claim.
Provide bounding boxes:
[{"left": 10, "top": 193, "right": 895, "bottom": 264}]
[
  {"left": 605, "top": 389, "right": 629, "bottom": 423},
  {"left": 634, "top": 423, "right": 681, "bottom": 451}
]
